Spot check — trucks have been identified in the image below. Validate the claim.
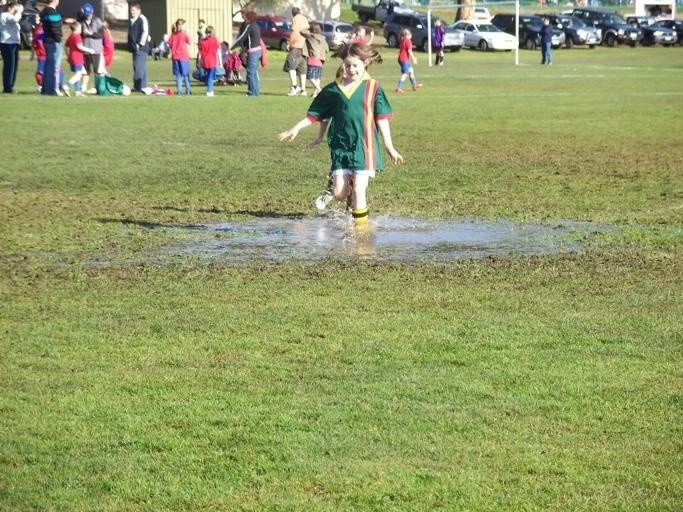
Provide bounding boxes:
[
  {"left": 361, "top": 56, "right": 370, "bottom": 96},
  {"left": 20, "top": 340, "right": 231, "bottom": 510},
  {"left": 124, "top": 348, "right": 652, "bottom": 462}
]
[{"left": 352, "top": 0, "right": 408, "bottom": 25}]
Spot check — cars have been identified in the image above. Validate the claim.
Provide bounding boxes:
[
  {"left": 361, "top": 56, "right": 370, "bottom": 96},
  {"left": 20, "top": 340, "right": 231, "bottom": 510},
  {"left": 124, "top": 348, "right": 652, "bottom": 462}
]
[
  {"left": 446, "top": 19, "right": 518, "bottom": 52},
  {"left": 473, "top": 6, "right": 490, "bottom": 23},
  {"left": 237, "top": 11, "right": 292, "bottom": 52},
  {"left": 650, "top": 19, "right": 683, "bottom": 46},
  {"left": 0, "top": 4, "right": 40, "bottom": 49}
]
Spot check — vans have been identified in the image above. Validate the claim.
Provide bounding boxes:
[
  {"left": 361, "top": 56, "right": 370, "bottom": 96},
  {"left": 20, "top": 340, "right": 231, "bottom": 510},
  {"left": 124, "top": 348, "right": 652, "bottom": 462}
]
[{"left": 307, "top": 20, "right": 354, "bottom": 49}]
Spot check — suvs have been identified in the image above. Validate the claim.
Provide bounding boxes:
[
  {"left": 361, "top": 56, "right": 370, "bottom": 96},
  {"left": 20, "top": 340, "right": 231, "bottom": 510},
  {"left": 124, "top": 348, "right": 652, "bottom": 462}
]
[
  {"left": 382, "top": 10, "right": 464, "bottom": 52},
  {"left": 533, "top": 13, "right": 601, "bottom": 48},
  {"left": 488, "top": 13, "right": 565, "bottom": 50},
  {"left": 571, "top": 7, "right": 643, "bottom": 47}
]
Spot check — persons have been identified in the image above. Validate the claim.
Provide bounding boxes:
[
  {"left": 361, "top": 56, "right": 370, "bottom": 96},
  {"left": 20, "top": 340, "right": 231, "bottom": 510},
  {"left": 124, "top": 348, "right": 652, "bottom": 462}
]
[
  {"left": 354, "top": 25, "right": 374, "bottom": 46},
  {"left": 307, "top": 65, "right": 366, "bottom": 213},
  {"left": 282, "top": 7, "right": 329, "bottom": 96},
  {"left": 539, "top": 18, "right": 554, "bottom": 65},
  {"left": 168, "top": 19, "right": 192, "bottom": 96},
  {"left": 395, "top": 28, "right": 423, "bottom": 94},
  {"left": 148, "top": 33, "right": 169, "bottom": 61},
  {"left": 434, "top": 19, "right": 446, "bottom": 66},
  {"left": 1, "top": 1, "right": 24, "bottom": 95},
  {"left": 32, "top": 1, "right": 114, "bottom": 96},
  {"left": 127, "top": 1, "right": 149, "bottom": 92},
  {"left": 193, "top": 11, "right": 263, "bottom": 96},
  {"left": 276, "top": 39, "right": 404, "bottom": 231}
]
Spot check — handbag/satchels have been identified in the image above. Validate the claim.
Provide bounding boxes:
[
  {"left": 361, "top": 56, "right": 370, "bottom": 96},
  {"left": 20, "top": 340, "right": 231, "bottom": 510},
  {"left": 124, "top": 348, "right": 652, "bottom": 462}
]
[{"left": 96, "top": 76, "right": 123, "bottom": 95}]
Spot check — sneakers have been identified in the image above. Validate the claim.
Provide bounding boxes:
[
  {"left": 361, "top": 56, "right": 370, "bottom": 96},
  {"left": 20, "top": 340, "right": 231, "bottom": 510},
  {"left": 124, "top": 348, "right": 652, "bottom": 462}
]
[
  {"left": 315, "top": 191, "right": 334, "bottom": 209},
  {"left": 51, "top": 84, "right": 82, "bottom": 96},
  {"left": 413, "top": 84, "right": 422, "bottom": 90},
  {"left": 287, "top": 86, "right": 321, "bottom": 96},
  {"left": 396, "top": 88, "right": 404, "bottom": 92}
]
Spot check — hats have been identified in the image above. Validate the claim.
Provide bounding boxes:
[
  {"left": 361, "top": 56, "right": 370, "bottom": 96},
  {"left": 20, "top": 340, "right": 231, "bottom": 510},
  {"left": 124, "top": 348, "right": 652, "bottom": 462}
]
[{"left": 83, "top": 3, "right": 92, "bottom": 15}]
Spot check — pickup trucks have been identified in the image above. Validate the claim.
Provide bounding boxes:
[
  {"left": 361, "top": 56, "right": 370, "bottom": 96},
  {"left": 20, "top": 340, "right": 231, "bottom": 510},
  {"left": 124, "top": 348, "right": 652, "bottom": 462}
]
[{"left": 624, "top": 15, "right": 677, "bottom": 46}]
